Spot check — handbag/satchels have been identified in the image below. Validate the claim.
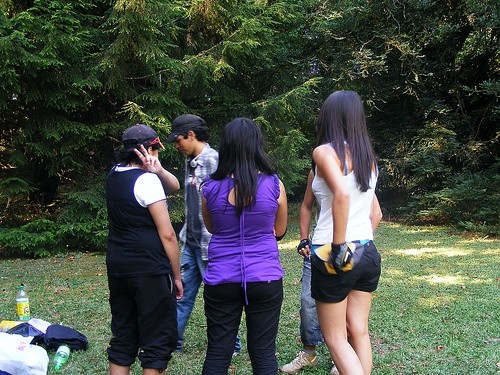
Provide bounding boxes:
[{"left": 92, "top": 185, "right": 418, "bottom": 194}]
[
  {"left": 0, "top": 331, "right": 49, "bottom": 375},
  {"left": 6, "top": 322, "right": 89, "bottom": 351}
]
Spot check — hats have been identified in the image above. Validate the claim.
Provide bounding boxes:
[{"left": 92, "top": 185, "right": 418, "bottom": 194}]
[
  {"left": 123, "top": 124, "right": 166, "bottom": 152},
  {"left": 165, "top": 114, "right": 205, "bottom": 142}
]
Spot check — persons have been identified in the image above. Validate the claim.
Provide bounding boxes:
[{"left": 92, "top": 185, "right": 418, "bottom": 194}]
[
  {"left": 165, "top": 114, "right": 241, "bottom": 356},
  {"left": 312, "top": 91, "right": 380, "bottom": 375},
  {"left": 282, "top": 155, "right": 383, "bottom": 375},
  {"left": 106, "top": 123, "right": 184, "bottom": 375},
  {"left": 201, "top": 116, "right": 288, "bottom": 375}
]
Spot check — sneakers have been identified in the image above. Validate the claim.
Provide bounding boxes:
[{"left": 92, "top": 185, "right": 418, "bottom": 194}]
[
  {"left": 330, "top": 360, "right": 341, "bottom": 375},
  {"left": 280, "top": 350, "right": 319, "bottom": 374}
]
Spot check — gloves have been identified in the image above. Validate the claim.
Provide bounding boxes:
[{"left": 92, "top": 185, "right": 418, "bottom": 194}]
[{"left": 329, "top": 242, "right": 353, "bottom": 274}]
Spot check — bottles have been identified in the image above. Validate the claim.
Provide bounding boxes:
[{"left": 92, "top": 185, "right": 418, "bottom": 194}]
[
  {"left": 16, "top": 286, "right": 30, "bottom": 321},
  {"left": 53, "top": 343, "right": 71, "bottom": 371}
]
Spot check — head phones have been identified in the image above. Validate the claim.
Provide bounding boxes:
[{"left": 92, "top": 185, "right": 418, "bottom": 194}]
[{"left": 186, "top": 142, "right": 210, "bottom": 168}]
